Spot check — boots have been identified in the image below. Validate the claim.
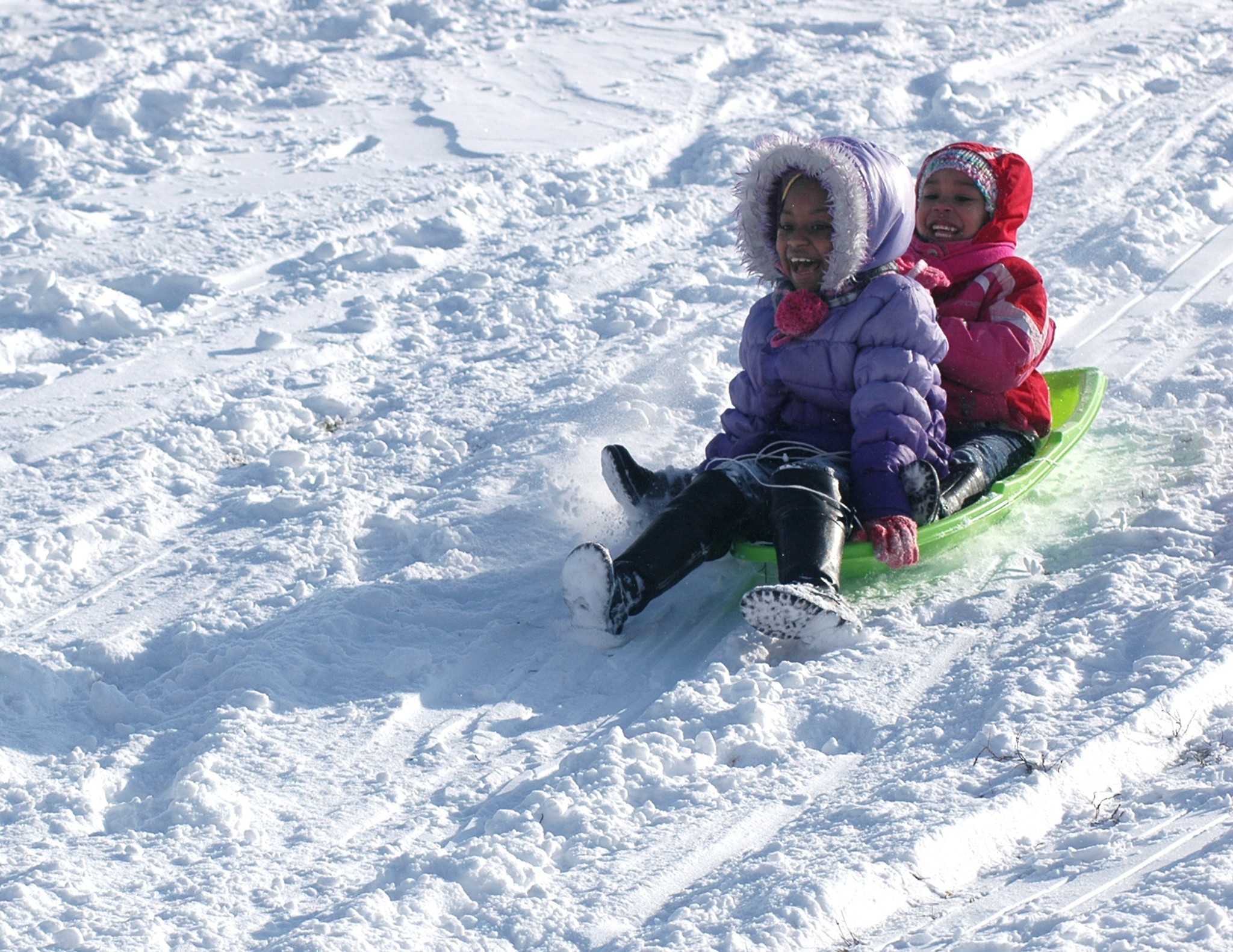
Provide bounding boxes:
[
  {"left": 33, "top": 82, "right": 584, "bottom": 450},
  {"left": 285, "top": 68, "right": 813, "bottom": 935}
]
[
  {"left": 738, "top": 469, "right": 862, "bottom": 649},
  {"left": 899, "top": 460, "right": 985, "bottom": 529},
  {"left": 560, "top": 470, "right": 745, "bottom": 636},
  {"left": 600, "top": 444, "right": 691, "bottom": 521}
]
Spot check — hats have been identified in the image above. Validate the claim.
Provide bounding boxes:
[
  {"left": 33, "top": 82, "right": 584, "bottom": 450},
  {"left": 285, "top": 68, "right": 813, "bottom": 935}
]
[{"left": 917, "top": 148, "right": 997, "bottom": 217}]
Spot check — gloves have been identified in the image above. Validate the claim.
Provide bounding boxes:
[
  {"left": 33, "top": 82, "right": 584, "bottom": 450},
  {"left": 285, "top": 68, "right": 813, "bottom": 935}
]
[{"left": 855, "top": 514, "right": 919, "bottom": 567}]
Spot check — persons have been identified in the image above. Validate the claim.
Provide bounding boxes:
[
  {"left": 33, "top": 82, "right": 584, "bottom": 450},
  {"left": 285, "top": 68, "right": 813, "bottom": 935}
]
[
  {"left": 600, "top": 142, "right": 1059, "bottom": 526},
  {"left": 561, "top": 136, "right": 949, "bottom": 641}
]
